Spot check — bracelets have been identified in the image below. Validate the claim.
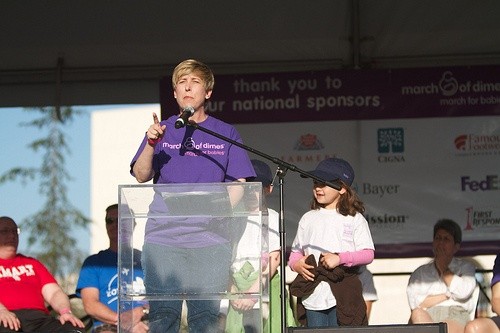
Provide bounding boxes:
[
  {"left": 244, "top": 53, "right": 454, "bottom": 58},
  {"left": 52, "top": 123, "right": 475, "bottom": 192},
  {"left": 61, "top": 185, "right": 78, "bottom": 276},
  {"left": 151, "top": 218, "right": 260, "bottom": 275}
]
[
  {"left": 147, "top": 130, "right": 165, "bottom": 146},
  {"left": 142, "top": 306, "right": 148, "bottom": 317},
  {"left": 59, "top": 308, "right": 72, "bottom": 315}
]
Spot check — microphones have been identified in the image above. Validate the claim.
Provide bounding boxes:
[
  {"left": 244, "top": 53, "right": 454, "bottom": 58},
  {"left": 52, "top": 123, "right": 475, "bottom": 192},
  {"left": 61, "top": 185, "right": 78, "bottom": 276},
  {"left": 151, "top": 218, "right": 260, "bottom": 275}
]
[{"left": 175, "top": 106, "right": 195, "bottom": 129}]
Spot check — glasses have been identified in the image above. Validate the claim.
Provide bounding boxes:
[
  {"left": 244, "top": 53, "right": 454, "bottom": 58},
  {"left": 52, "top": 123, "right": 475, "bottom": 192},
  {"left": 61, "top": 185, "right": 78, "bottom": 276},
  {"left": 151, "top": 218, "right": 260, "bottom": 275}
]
[
  {"left": 106, "top": 218, "right": 118, "bottom": 224},
  {"left": 1, "top": 228, "right": 21, "bottom": 235}
]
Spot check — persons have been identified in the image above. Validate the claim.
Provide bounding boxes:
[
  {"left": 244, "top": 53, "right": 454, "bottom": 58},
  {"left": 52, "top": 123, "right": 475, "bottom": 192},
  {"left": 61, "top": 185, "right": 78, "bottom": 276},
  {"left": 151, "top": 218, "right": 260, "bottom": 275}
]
[
  {"left": 130, "top": 58, "right": 255, "bottom": 333},
  {"left": 0, "top": 157, "right": 379, "bottom": 333},
  {"left": 406, "top": 218, "right": 500, "bottom": 333}
]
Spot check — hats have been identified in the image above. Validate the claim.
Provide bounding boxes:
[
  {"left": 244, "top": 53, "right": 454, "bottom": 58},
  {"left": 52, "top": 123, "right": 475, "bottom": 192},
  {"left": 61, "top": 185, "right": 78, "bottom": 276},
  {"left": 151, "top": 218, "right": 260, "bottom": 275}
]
[
  {"left": 300, "top": 158, "right": 354, "bottom": 186},
  {"left": 246, "top": 159, "right": 272, "bottom": 189}
]
